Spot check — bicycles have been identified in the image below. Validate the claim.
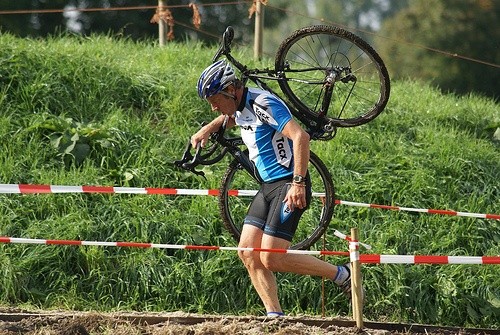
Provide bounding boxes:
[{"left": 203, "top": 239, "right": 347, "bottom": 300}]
[{"left": 174, "top": 24, "right": 390, "bottom": 251}]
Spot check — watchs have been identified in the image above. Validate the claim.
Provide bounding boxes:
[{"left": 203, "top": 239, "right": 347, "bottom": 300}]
[{"left": 292, "top": 174, "right": 307, "bottom": 183}]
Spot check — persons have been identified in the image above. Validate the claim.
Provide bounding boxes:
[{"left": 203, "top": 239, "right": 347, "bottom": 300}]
[{"left": 189, "top": 57, "right": 353, "bottom": 327}]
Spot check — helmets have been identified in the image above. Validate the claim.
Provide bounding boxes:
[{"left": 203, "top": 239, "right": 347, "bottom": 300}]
[{"left": 197, "top": 58, "right": 238, "bottom": 100}]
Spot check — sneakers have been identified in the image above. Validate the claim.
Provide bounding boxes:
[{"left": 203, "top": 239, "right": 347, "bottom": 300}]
[{"left": 340, "top": 262, "right": 364, "bottom": 304}]
